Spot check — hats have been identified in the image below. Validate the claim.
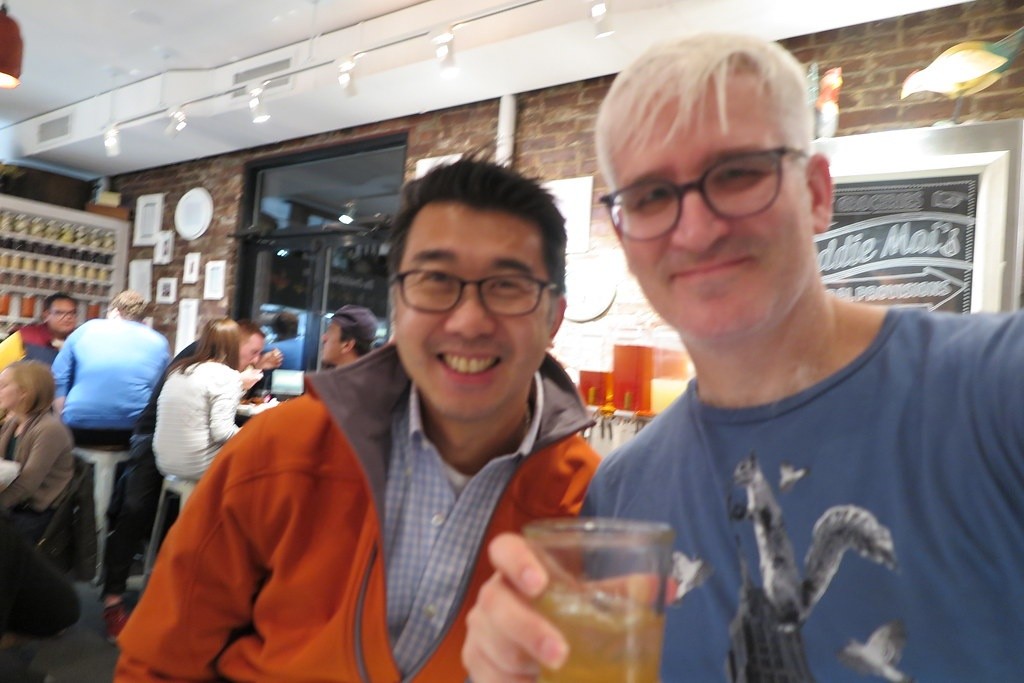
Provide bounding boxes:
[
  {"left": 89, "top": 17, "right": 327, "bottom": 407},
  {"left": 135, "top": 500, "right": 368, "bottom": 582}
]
[{"left": 333, "top": 304, "right": 378, "bottom": 345}]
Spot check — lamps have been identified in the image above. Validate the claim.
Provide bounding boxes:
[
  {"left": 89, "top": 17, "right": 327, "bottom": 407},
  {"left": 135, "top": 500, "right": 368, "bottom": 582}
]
[
  {"left": 430, "top": 25, "right": 454, "bottom": 61},
  {"left": 337, "top": 53, "right": 357, "bottom": 96},
  {"left": 246, "top": 83, "right": 270, "bottom": 123},
  {"left": 103, "top": 124, "right": 121, "bottom": 158},
  {"left": 591, "top": 0, "right": 615, "bottom": 38},
  {"left": 163, "top": 109, "right": 184, "bottom": 142}
]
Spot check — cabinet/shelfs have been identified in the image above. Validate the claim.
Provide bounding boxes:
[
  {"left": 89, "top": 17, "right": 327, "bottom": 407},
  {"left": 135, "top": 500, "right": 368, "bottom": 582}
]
[{"left": 0, "top": 195, "right": 131, "bottom": 343}]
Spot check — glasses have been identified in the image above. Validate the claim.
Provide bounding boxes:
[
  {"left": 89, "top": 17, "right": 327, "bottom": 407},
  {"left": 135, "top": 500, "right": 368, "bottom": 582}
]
[
  {"left": 48, "top": 311, "right": 78, "bottom": 320},
  {"left": 389, "top": 267, "right": 559, "bottom": 317},
  {"left": 600, "top": 148, "right": 812, "bottom": 241}
]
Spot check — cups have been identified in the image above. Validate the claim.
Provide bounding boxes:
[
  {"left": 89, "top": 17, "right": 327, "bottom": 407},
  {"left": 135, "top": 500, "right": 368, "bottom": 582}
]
[{"left": 521, "top": 516, "right": 673, "bottom": 682}]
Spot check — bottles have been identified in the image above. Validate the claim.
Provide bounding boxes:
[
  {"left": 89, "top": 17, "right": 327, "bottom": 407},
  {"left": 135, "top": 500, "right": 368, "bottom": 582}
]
[{"left": 0, "top": 213, "right": 116, "bottom": 323}]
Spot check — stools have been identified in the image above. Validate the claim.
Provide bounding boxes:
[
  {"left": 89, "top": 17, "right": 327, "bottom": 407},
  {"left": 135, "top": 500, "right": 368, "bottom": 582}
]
[
  {"left": 65, "top": 445, "right": 131, "bottom": 586},
  {"left": 122, "top": 474, "right": 195, "bottom": 592}
]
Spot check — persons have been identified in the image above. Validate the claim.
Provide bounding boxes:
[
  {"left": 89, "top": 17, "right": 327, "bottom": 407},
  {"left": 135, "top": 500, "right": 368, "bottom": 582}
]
[
  {"left": 0, "top": 292, "right": 77, "bottom": 371},
  {"left": 460, "top": 31, "right": 1024, "bottom": 683},
  {"left": 262, "top": 311, "right": 302, "bottom": 369},
  {"left": 114, "top": 160, "right": 603, "bottom": 682},
  {"left": 0, "top": 363, "right": 73, "bottom": 547},
  {"left": 46, "top": 293, "right": 171, "bottom": 448},
  {"left": 321, "top": 305, "right": 376, "bottom": 368},
  {"left": 102, "top": 323, "right": 285, "bottom": 646},
  {"left": 154, "top": 319, "right": 255, "bottom": 483}
]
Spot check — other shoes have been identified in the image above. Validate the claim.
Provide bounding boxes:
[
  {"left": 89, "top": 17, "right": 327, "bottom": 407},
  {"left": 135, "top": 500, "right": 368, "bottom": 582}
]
[{"left": 104, "top": 601, "right": 128, "bottom": 645}]
